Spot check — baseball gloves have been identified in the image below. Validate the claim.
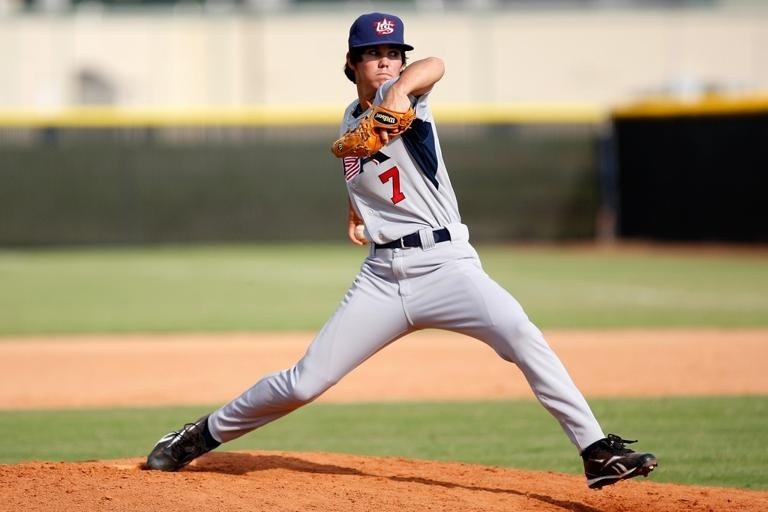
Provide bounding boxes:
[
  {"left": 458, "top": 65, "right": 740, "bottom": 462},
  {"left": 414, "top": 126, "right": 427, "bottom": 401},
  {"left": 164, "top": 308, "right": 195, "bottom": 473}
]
[{"left": 332, "top": 106, "right": 416, "bottom": 159}]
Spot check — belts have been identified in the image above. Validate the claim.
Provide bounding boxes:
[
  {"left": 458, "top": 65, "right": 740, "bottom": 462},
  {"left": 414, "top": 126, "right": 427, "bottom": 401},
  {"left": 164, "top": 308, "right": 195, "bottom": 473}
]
[{"left": 373, "top": 228, "right": 452, "bottom": 251}]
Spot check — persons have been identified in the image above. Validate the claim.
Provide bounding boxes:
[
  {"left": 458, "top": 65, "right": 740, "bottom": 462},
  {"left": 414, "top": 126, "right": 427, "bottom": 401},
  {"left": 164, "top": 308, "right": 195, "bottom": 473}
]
[{"left": 146, "top": 12, "right": 658, "bottom": 489}]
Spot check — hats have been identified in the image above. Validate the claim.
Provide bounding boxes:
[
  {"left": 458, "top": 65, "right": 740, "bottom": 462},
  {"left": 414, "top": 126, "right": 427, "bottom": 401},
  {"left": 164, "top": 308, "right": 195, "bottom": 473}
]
[{"left": 347, "top": 11, "right": 414, "bottom": 53}]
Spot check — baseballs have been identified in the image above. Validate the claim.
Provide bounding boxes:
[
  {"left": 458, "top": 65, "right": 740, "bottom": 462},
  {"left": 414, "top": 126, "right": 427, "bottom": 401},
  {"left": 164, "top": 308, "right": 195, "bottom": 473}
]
[{"left": 354, "top": 225, "right": 371, "bottom": 244}]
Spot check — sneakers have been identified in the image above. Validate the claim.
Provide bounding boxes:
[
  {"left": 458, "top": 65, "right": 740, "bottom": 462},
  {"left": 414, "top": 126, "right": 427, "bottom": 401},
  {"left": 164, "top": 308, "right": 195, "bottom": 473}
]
[
  {"left": 582, "top": 436, "right": 657, "bottom": 490},
  {"left": 146, "top": 413, "right": 223, "bottom": 471}
]
